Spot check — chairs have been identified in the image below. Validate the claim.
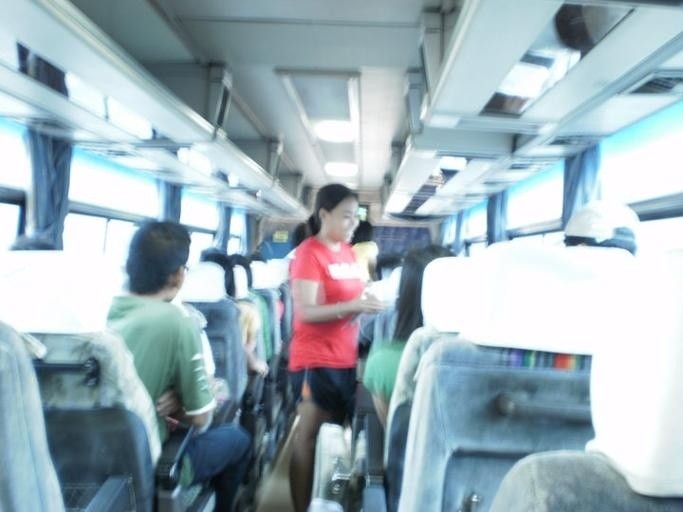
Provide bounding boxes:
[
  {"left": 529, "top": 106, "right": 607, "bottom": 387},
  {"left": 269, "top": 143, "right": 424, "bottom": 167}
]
[
  {"left": 0, "top": 246, "right": 286, "bottom": 512},
  {"left": 364, "top": 255, "right": 683, "bottom": 512}
]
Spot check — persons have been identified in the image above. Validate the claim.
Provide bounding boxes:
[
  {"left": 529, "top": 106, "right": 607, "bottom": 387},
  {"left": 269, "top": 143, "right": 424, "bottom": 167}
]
[
  {"left": 564, "top": 200, "right": 643, "bottom": 255},
  {"left": 199, "top": 251, "right": 273, "bottom": 377},
  {"left": 351, "top": 221, "right": 379, "bottom": 275},
  {"left": 285, "top": 180, "right": 390, "bottom": 512},
  {"left": 362, "top": 244, "right": 456, "bottom": 425},
  {"left": 357, "top": 249, "right": 403, "bottom": 347},
  {"left": 105, "top": 219, "right": 251, "bottom": 512}
]
